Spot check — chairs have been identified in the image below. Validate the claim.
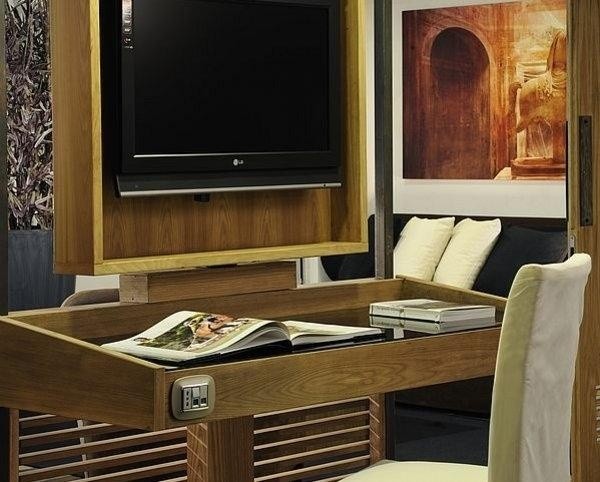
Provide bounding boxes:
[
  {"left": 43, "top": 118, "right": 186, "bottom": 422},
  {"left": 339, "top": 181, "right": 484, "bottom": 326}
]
[{"left": 341, "top": 250, "right": 593, "bottom": 482}]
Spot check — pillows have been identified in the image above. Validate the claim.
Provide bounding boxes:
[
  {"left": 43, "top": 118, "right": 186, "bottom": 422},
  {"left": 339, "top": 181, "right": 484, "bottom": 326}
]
[{"left": 391, "top": 215, "right": 568, "bottom": 299}]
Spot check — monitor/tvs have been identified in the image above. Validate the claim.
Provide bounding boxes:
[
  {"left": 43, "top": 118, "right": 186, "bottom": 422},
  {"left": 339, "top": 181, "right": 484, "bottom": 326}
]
[{"left": 98, "top": 0, "right": 343, "bottom": 202}]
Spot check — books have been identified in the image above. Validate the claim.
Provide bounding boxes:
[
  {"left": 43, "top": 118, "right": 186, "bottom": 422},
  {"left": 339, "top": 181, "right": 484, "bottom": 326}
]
[
  {"left": 101, "top": 310, "right": 385, "bottom": 367},
  {"left": 368, "top": 315, "right": 495, "bottom": 335},
  {"left": 369, "top": 297, "right": 496, "bottom": 322}
]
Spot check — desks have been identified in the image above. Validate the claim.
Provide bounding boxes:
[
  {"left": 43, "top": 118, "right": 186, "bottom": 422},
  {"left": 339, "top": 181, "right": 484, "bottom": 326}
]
[{"left": 0, "top": 275, "right": 509, "bottom": 481}]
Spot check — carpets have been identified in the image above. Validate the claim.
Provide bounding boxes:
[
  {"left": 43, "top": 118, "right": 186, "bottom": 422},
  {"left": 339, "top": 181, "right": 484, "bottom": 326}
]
[{"left": 396, "top": 410, "right": 484, "bottom": 445}]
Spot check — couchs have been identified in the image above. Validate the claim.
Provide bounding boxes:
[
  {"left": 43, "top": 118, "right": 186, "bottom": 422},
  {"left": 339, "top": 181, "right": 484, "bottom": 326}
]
[{"left": 321, "top": 212, "right": 568, "bottom": 416}]
